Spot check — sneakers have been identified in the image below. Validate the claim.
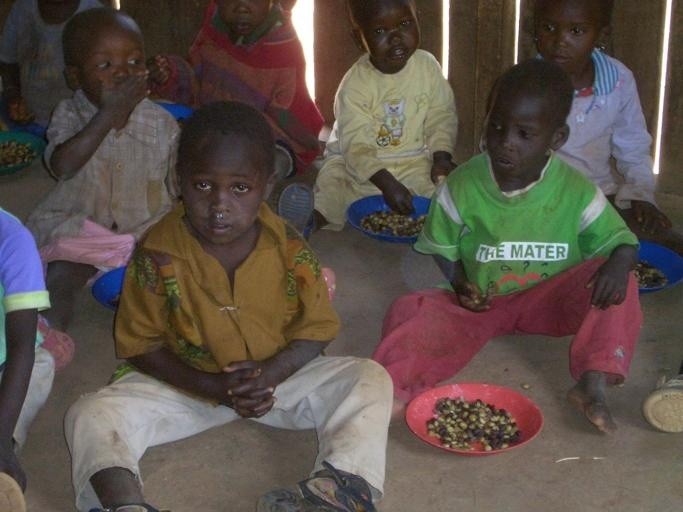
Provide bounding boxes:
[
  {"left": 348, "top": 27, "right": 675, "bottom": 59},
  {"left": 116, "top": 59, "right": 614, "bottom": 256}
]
[
  {"left": 277, "top": 183, "right": 315, "bottom": 239},
  {"left": 38, "top": 315, "right": 74, "bottom": 368},
  {"left": 642, "top": 373, "right": 683, "bottom": 434},
  {"left": 257, "top": 460, "right": 375, "bottom": 511}
]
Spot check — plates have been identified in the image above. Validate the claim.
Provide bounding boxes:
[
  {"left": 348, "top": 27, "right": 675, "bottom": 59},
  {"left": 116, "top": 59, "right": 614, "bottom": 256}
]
[
  {"left": 344, "top": 192, "right": 431, "bottom": 245},
  {"left": 91, "top": 264, "right": 126, "bottom": 313},
  {"left": 154, "top": 103, "right": 193, "bottom": 121},
  {"left": 626, "top": 239, "right": 682, "bottom": 296},
  {"left": 0, "top": 130, "right": 47, "bottom": 177},
  {"left": 402, "top": 383, "right": 546, "bottom": 459}
]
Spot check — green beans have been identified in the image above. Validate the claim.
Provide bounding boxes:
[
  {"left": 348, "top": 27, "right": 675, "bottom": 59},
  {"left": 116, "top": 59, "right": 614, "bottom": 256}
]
[
  {"left": 1, "top": 134, "right": 39, "bottom": 171},
  {"left": 425, "top": 396, "right": 521, "bottom": 452},
  {"left": 359, "top": 209, "right": 430, "bottom": 241},
  {"left": 632, "top": 259, "right": 669, "bottom": 290}
]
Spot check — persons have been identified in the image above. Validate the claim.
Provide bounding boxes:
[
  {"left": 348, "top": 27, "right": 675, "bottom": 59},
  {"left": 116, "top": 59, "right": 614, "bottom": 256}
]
[
  {"left": 26, "top": 8, "right": 181, "bottom": 334},
  {"left": 371, "top": 58, "right": 644, "bottom": 434},
  {"left": 0, "top": 0, "right": 107, "bottom": 130},
  {"left": 68, "top": 99, "right": 394, "bottom": 512},
  {"left": 1, "top": 207, "right": 57, "bottom": 512},
  {"left": 479, "top": 0, "right": 683, "bottom": 258},
  {"left": 146, "top": 0, "right": 326, "bottom": 182},
  {"left": 280, "top": 0, "right": 460, "bottom": 235}
]
